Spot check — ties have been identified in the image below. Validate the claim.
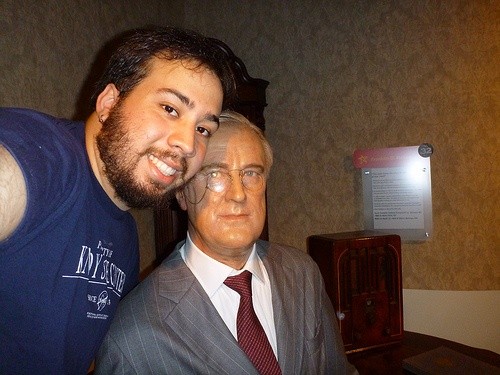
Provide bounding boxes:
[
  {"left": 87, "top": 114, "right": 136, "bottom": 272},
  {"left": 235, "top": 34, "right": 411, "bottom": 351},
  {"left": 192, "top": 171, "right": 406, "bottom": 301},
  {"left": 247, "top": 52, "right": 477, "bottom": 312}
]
[{"left": 224, "top": 271, "right": 282, "bottom": 375}]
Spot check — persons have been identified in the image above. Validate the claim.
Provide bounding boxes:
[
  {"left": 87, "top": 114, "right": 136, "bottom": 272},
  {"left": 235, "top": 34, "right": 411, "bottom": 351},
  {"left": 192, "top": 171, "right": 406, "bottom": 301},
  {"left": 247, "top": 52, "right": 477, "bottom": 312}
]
[
  {"left": 95, "top": 110, "right": 346, "bottom": 375},
  {"left": 1, "top": 27, "right": 237, "bottom": 375}
]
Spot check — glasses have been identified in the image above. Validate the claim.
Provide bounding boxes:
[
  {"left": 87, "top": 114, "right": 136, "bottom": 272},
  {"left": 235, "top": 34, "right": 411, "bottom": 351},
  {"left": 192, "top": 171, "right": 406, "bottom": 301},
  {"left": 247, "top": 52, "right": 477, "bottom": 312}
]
[{"left": 191, "top": 170, "right": 269, "bottom": 193}]
